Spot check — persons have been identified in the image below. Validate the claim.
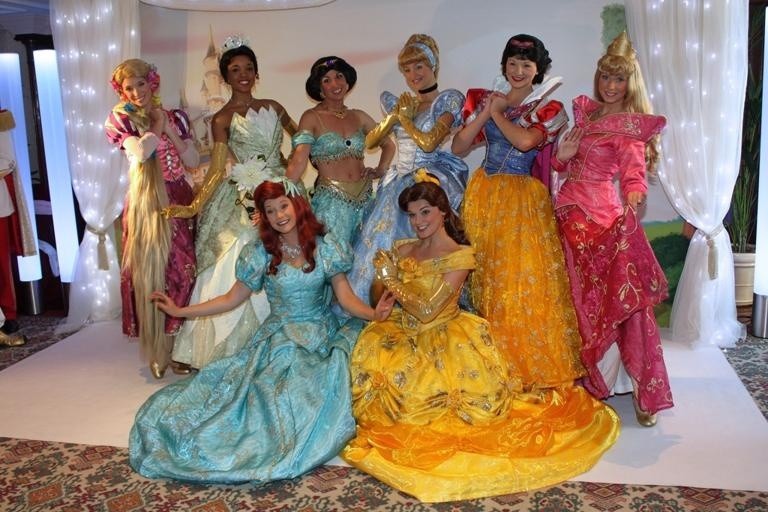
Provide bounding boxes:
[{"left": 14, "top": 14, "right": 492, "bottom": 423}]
[
  {"left": 0, "top": 92, "right": 27, "bottom": 348},
  {"left": 452, "top": 35, "right": 588, "bottom": 390},
  {"left": 103, "top": 60, "right": 202, "bottom": 380},
  {"left": 129, "top": 180, "right": 396, "bottom": 485},
  {"left": 336, "top": 34, "right": 470, "bottom": 323},
  {"left": 161, "top": 45, "right": 300, "bottom": 383},
  {"left": 284, "top": 56, "right": 396, "bottom": 265},
  {"left": 547, "top": 30, "right": 675, "bottom": 427},
  {"left": 339, "top": 172, "right": 620, "bottom": 505}
]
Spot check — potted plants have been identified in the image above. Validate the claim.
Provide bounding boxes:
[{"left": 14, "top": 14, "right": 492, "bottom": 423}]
[{"left": 724, "top": 0, "right": 765, "bottom": 306}]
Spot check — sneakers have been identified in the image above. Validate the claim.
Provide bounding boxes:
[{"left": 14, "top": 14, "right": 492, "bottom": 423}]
[{"left": 4, "top": 320, "right": 19, "bottom": 334}]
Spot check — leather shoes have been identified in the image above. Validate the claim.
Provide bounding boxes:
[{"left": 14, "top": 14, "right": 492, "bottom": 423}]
[
  {"left": 632, "top": 391, "right": 656, "bottom": 426},
  {"left": 171, "top": 360, "right": 191, "bottom": 374},
  {"left": 150, "top": 356, "right": 165, "bottom": 378}
]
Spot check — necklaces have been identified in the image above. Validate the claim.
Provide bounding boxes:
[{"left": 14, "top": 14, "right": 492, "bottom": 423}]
[
  {"left": 417, "top": 84, "right": 442, "bottom": 96},
  {"left": 232, "top": 97, "right": 256, "bottom": 107},
  {"left": 275, "top": 234, "right": 307, "bottom": 260},
  {"left": 325, "top": 105, "right": 350, "bottom": 120}
]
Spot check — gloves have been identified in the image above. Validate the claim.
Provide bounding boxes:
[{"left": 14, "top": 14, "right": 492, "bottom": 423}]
[
  {"left": 397, "top": 90, "right": 450, "bottom": 152},
  {"left": 371, "top": 247, "right": 456, "bottom": 324},
  {"left": 371, "top": 249, "right": 399, "bottom": 305},
  {"left": 363, "top": 92, "right": 418, "bottom": 150},
  {"left": 159, "top": 142, "right": 228, "bottom": 221}
]
